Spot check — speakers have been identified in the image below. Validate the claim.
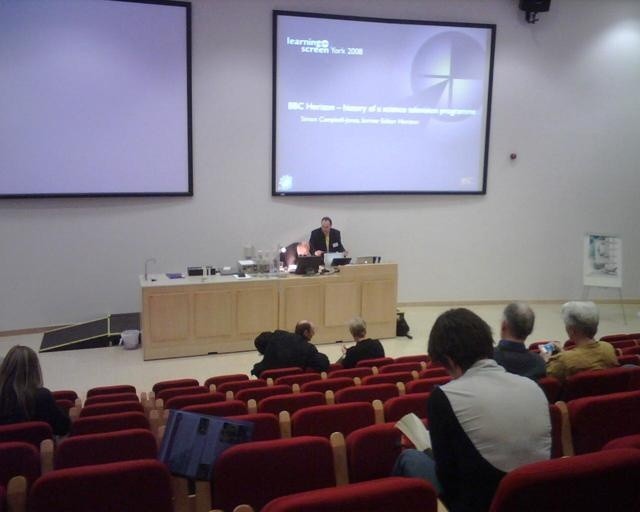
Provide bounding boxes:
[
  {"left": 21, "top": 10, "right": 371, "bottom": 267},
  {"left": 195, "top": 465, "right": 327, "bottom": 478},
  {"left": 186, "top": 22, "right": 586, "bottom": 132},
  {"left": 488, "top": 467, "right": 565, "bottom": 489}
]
[{"left": 519, "top": 0, "right": 551, "bottom": 12}]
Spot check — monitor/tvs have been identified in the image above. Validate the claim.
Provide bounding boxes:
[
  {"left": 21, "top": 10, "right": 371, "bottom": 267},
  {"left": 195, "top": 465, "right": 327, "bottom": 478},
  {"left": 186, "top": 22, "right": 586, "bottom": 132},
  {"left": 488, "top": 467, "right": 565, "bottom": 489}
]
[
  {"left": 355, "top": 256, "right": 377, "bottom": 264},
  {"left": 330, "top": 258, "right": 351, "bottom": 266}
]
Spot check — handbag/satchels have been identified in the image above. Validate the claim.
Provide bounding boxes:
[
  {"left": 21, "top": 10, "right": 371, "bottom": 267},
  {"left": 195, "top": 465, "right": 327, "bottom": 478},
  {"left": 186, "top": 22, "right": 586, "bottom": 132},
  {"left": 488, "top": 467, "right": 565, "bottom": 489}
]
[{"left": 396, "top": 317, "right": 412, "bottom": 339}]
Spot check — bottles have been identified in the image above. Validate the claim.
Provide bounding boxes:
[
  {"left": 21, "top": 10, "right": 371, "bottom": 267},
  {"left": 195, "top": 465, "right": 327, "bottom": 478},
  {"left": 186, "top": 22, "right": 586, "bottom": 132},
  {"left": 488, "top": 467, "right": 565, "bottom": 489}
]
[{"left": 257, "top": 250, "right": 280, "bottom": 275}]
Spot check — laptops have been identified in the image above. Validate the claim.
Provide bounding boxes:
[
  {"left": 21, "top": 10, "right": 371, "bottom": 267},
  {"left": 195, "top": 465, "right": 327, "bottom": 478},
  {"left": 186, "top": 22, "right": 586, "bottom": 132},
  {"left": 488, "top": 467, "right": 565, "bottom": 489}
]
[{"left": 289, "top": 257, "right": 320, "bottom": 274}]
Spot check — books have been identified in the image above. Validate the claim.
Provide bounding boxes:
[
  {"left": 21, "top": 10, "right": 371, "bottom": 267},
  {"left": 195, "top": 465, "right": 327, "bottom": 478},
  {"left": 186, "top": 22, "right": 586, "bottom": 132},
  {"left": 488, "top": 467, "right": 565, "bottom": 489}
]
[{"left": 394, "top": 409, "right": 432, "bottom": 455}]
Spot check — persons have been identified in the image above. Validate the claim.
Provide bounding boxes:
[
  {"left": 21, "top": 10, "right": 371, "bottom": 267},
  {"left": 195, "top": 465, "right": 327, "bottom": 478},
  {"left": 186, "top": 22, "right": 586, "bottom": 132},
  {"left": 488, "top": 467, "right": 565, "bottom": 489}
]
[
  {"left": 247, "top": 318, "right": 330, "bottom": 379},
  {"left": 395, "top": 305, "right": 555, "bottom": 511},
  {"left": 488, "top": 301, "right": 548, "bottom": 381},
  {"left": 309, "top": 216, "right": 348, "bottom": 259},
  {"left": 279, "top": 240, "right": 313, "bottom": 274},
  {"left": 539, "top": 299, "right": 620, "bottom": 382},
  {"left": 0, "top": 343, "right": 72, "bottom": 437},
  {"left": 343, "top": 318, "right": 387, "bottom": 368}
]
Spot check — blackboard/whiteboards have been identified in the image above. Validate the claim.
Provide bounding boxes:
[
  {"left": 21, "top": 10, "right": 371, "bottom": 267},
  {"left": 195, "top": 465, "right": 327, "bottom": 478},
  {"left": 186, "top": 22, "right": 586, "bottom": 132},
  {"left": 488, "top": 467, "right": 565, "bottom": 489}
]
[
  {"left": 109, "top": 312, "right": 141, "bottom": 335},
  {"left": 39, "top": 315, "right": 109, "bottom": 354}
]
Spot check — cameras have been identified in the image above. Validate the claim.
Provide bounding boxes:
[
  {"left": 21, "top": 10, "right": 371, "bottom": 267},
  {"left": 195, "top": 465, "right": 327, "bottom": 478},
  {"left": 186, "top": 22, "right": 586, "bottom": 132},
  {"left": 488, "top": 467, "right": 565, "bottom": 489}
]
[{"left": 538, "top": 342, "right": 556, "bottom": 353}]
